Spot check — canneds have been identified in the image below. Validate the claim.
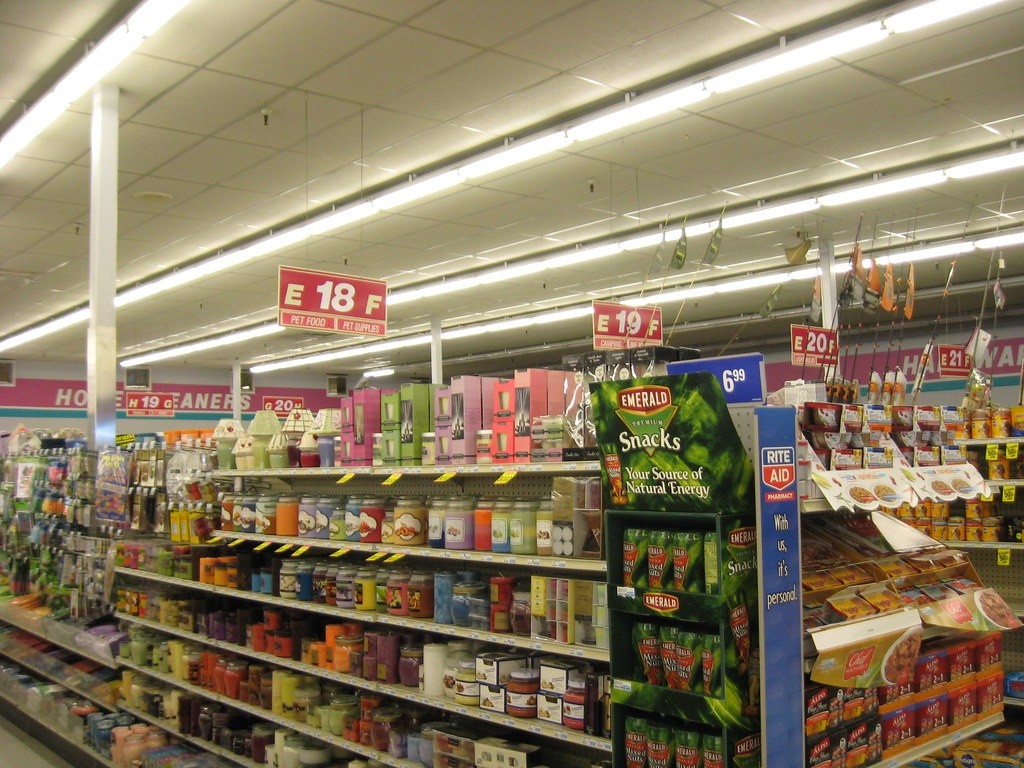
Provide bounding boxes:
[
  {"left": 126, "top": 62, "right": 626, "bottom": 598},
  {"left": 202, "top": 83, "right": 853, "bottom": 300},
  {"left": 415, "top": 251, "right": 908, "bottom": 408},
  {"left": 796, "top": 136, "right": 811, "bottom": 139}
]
[{"left": 880, "top": 404, "right": 1024, "bottom": 541}]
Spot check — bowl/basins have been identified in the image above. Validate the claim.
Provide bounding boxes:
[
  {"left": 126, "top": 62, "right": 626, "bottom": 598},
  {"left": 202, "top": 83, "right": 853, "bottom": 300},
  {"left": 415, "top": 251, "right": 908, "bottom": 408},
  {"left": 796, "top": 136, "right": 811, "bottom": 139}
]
[
  {"left": 930, "top": 479, "right": 955, "bottom": 497},
  {"left": 950, "top": 477, "right": 975, "bottom": 496},
  {"left": 848, "top": 485, "right": 875, "bottom": 508},
  {"left": 872, "top": 483, "right": 899, "bottom": 504},
  {"left": 974, "top": 591, "right": 1010, "bottom": 630},
  {"left": 881, "top": 625, "right": 923, "bottom": 684}
]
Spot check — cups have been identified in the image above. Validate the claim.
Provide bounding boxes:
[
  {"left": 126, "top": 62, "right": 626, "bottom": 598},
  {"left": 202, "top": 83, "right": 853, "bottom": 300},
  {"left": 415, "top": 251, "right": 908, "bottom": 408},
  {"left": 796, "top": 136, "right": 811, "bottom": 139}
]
[
  {"left": 88, "top": 492, "right": 585, "bottom": 768},
  {"left": 421, "top": 432, "right": 435, "bottom": 465},
  {"left": 372, "top": 432, "right": 383, "bottom": 466},
  {"left": 475, "top": 429, "right": 492, "bottom": 464}
]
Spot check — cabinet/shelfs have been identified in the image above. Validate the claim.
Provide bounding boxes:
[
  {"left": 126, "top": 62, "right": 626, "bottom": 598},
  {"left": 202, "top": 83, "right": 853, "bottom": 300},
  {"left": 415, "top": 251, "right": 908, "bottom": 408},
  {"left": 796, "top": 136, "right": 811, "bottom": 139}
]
[
  {"left": 2, "top": 428, "right": 212, "bottom": 762},
  {"left": 875, "top": 402, "right": 1024, "bottom": 767},
  {"left": 109, "top": 350, "right": 1007, "bottom": 767}
]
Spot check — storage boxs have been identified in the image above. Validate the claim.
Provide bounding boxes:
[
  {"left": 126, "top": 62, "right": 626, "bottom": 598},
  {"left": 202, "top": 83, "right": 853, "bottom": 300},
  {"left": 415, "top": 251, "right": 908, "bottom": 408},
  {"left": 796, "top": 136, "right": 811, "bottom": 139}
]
[
  {"left": 904, "top": 726, "right": 1024, "bottom": 768},
  {"left": 337, "top": 369, "right": 584, "bottom": 469},
  {"left": 432, "top": 646, "right": 592, "bottom": 768},
  {"left": 799, "top": 515, "right": 1004, "bottom": 768}
]
